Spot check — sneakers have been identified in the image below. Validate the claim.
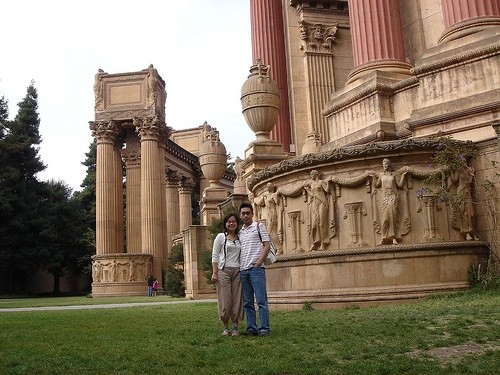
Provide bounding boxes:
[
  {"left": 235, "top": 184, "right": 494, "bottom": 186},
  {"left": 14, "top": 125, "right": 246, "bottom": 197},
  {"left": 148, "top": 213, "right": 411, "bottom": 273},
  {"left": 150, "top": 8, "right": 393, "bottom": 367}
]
[
  {"left": 245, "top": 329, "right": 257, "bottom": 336},
  {"left": 260, "top": 329, "right": 270, "bottom": 336},
  {"left": 231, "top": 330, "right": 239, "bottom": 336},
  {"left": 222, "top": 330, "right": 229, "bottom": 336}
]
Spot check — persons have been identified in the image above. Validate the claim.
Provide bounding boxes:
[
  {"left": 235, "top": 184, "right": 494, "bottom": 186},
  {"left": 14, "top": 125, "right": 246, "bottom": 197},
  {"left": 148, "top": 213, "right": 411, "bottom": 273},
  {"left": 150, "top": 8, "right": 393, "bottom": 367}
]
[
  {"left": 108, "top": 260, "right": 118, "bottom": 281},
  {"left": 263, "top": 183, "right": 283, "bottom": 256},
  {"left": 303, "top": 170, "right": 332, "bottom": 251},
  {"left": 148, "top": 274, "right": 159, "bottom": 296},
  {"left": 144, "top": 258, "right": 152, "bottom": 280},
  {"left": 211, "top": 214, "right": 243, "bottom": 336},
  {"left": 128, "top": 259, "right": 137, "bottom": 281},
  {"left": 238, "top": 203, "right": 270, "bottom": 336},
  {"left": 369, "top": 159, "right": 407, "bottom": 245},
  {"left": 446, "top": 159, "right": 481, "bottom": 241},
  {"left": 94, "top": 261, "right": 104, "bottom": 282}
]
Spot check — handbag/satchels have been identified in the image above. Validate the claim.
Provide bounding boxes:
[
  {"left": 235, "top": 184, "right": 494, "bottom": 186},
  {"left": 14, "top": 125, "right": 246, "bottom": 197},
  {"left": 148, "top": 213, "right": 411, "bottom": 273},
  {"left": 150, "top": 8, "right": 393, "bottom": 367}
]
[{"left": 262, "top": 238, "right": 277, "bottom": 265}]
